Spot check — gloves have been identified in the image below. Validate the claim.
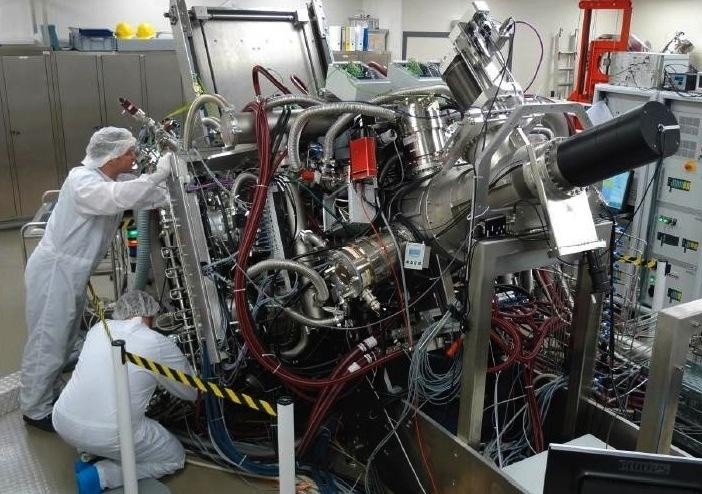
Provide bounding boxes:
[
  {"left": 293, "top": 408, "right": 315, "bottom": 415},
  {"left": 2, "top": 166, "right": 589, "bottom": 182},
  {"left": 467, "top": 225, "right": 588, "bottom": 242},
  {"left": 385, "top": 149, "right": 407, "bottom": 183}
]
[{"left": 148, "top": 153, "right": 172, "bottom": 186}]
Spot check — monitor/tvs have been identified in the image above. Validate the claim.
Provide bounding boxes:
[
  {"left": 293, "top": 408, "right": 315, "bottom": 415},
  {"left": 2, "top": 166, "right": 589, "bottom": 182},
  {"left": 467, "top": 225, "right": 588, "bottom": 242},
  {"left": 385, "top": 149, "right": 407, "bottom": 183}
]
[{"left": 543, "top": 442, "right": 702, "bottom": 494}]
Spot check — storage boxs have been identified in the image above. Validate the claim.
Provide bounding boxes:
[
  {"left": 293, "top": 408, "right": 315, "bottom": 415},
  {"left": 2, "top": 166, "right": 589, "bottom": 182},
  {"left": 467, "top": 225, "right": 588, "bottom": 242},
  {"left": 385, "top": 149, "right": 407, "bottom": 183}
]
[{"left": 341, "top": 27, "right": 390, "bottom": 53}]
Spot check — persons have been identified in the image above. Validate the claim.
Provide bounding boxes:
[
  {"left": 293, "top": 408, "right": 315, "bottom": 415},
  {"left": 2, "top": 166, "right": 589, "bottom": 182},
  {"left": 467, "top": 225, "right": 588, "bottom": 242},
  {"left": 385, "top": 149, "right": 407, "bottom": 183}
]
[
  {"left": 17, "top": 125, "right": 173, "bottom": 431},
  {"left": 52, "top": 288, "right": 198, "bottom": 491}
]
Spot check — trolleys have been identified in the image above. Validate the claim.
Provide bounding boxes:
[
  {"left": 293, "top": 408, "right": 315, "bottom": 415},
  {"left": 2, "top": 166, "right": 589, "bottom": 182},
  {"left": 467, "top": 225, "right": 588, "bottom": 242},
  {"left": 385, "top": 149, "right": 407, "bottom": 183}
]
[{"left": 21, "top": 188, "right": 125, "bottom": 341}]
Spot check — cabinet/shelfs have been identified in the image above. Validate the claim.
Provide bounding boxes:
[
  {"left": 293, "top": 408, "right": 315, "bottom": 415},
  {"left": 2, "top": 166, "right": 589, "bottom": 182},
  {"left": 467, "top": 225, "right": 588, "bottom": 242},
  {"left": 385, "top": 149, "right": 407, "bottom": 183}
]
[
  {"left": 140, "top": 50, "right": 200, "bottom": 144},
  {"left": 51, "top": 51, "right": 144, "bottom": 196},
  {"left": 333, "top": 50, "right": 391, "bottom": 75},
  {"left": 0, "top": 43, "right": 63, "bottom": 234}
]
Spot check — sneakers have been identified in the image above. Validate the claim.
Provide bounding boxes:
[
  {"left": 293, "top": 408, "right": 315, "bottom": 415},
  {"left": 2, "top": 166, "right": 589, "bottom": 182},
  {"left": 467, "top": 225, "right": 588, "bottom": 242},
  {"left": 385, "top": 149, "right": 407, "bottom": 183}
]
[{"left": 23, "top": 414, "right": 55, "bottom": 433}]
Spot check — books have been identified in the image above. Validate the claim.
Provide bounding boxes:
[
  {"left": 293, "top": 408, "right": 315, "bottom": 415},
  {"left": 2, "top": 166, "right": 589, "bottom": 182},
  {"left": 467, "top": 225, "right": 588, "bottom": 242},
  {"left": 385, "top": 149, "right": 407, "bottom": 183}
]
[{"left": 330, "top": 24, "right": 368, "bottom": 52}]
